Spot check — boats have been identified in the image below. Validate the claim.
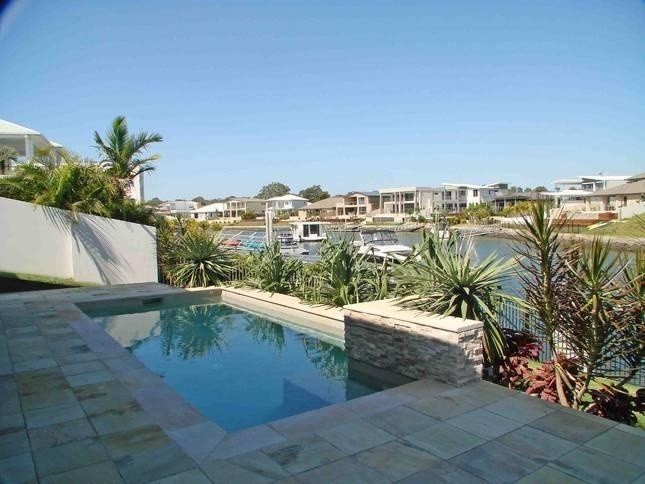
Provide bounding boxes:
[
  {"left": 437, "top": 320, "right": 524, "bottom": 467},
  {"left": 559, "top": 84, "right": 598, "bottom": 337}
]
[
  {"left": 427, "top": 221, "right": 461, "bottom": 245},
  {"left": 215, "top": 231, "right": 310, "bottom": 258},
  {"left": 349, "top": 226, "right": 428, "bottom": 269},
  {"left": 288, "top": 221, "right": 332, "bottom": 243}
]
